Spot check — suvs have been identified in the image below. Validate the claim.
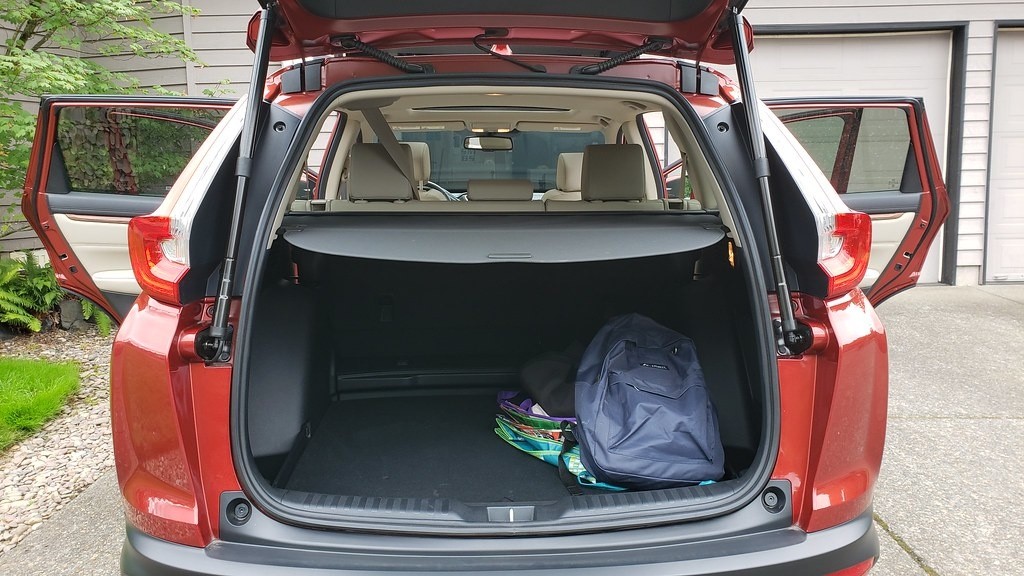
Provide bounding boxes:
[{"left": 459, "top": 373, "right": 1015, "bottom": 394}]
[{"left": 19, "top": 0, "right": 952, "bottom": 575}]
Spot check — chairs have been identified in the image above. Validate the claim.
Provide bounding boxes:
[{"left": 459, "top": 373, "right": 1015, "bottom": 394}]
[{"left": 326, "top": 140, "right": 674, "bottom": 215}]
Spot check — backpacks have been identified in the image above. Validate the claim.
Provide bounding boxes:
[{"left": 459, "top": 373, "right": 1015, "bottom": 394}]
[{"left": 556, "top": 312, "right": 727, "bottom": 495}]
